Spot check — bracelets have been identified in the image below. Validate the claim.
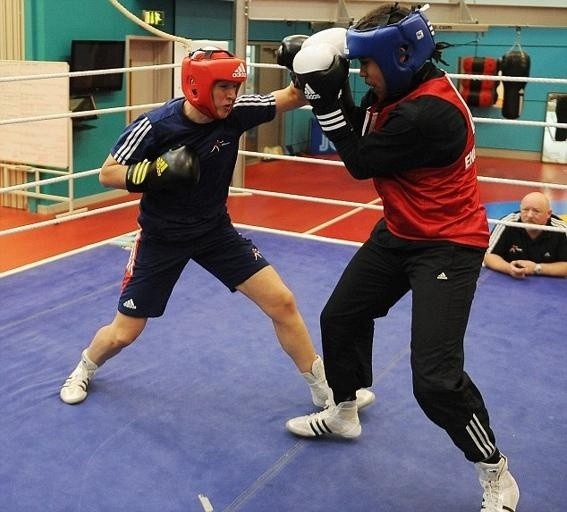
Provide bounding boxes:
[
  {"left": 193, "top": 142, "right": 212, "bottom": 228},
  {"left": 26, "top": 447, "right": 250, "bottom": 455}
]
[{"left": 535, "top": 263, "right": 543, "bottom": 276}]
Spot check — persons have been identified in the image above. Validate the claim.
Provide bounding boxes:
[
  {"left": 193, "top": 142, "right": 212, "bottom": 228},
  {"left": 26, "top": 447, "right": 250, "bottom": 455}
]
[
  {"left": 60, "top": 46, "right": 374, "bottom": 409},
  {"left": 276, "top": 4, "right": 520, "bottom": 511},
  {"left": 481, "top": 191, "right": 567, "bottom": 279}
]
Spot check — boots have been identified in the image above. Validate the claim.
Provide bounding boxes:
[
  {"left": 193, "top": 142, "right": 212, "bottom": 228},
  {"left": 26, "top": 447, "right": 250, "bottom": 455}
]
[
  {"left": 286, "top": 354, "right": 376, "bottom": 439},
  {"left": 60, "top": 348, "right": 105, "bottom": 404},
  {"left": 475, "top": 451, "right": 520, "bottom": 512}
]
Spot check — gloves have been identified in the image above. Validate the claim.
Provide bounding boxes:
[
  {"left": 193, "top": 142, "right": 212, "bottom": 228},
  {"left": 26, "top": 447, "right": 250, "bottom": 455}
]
[
  {"left": 126, "top": 144, "right": 209, "bottom": 197},
  {"left": 277, "top": 28, "right": 360, "bottom": 143}
]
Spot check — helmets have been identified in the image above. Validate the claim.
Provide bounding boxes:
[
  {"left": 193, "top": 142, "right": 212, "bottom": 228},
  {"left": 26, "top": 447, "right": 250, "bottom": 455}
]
[{"left": 182, "top": 46, "right": 247, "bottom": 120}]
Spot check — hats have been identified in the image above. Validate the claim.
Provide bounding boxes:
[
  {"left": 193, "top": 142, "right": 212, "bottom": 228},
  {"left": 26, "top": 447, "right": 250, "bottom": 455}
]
[{"left": 346, "top": 8, "right": 437, "bottom": 98}]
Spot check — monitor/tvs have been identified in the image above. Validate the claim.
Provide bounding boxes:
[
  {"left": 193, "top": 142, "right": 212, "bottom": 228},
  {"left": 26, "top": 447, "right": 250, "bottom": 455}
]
[{"left": 70, "top": 40, "right": 125, "bottom": 96}]
